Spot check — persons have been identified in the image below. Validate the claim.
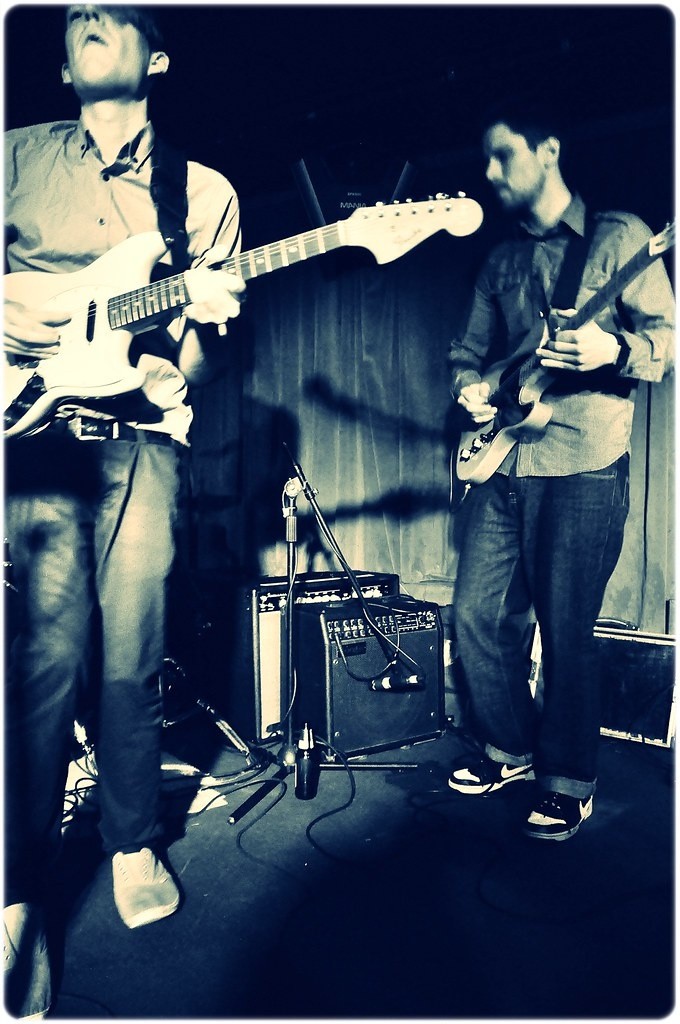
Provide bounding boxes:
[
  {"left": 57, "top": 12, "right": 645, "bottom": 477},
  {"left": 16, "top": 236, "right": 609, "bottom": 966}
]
[
  {"left": 447, "top": 105, "right": 678, "bottom": 841},
  {"left": 3, "top": 4, "right": 245, "bottom": 1018}
]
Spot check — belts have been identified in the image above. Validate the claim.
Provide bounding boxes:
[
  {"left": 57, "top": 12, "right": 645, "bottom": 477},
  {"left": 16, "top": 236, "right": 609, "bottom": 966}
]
[{"left": 64, "top": 413, "right": 175, "bottom": 446}]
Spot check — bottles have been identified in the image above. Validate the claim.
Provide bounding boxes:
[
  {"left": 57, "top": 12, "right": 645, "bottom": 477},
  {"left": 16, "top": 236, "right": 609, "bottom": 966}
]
[{"left": 295, "top": 722, "right": 319, "bottom": 800}]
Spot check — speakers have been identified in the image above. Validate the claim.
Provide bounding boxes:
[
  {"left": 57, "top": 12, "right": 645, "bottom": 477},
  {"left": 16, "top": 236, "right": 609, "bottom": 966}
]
[
  {"left": 230, "top": 571, "right": 403, "bottom": 745},
  {"left": 279, "top": 596, "right": 446, "bottom": 761}
]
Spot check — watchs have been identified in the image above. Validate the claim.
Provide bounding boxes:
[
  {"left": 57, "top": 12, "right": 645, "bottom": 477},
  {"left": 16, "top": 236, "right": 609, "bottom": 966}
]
[{"left": 612, "top": 332, "right": 634, "bottom": 371}]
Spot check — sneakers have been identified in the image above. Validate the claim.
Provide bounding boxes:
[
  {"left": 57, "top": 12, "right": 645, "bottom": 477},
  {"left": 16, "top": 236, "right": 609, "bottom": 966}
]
[
  {"left": 446, "top": 761, "right": 532, "bottom": 796},
  {"left": 523, "top": 791, "right": 596, "bottom": 842}
]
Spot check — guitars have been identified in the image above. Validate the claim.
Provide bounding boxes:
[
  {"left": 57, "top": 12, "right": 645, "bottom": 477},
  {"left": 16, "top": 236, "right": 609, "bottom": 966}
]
[
  {"left": 0, "top": 187, "right": 486, "bottom": 445},
  {"left": 455, "top": 219, "right": 675, "bottom": 484}
]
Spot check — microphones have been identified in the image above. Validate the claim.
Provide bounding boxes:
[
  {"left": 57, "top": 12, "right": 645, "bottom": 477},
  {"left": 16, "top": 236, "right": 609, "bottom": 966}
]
[{"left": 370, "top": 675, "right": 428, "bottom": 691}]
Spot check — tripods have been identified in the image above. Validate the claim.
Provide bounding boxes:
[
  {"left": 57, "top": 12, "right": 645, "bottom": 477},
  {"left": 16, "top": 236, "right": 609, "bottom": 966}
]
[{"left": 154, "top": 442, "right": 438, "bottom": 823}]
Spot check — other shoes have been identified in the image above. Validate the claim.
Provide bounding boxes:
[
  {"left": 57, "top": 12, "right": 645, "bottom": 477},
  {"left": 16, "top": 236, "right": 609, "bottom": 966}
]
[
  {"left": 110, "top": 846, "right": 180, "bottom": 930},
  {"left": 3, "top": 904, "right": 54, "bottom": 1022}
]
